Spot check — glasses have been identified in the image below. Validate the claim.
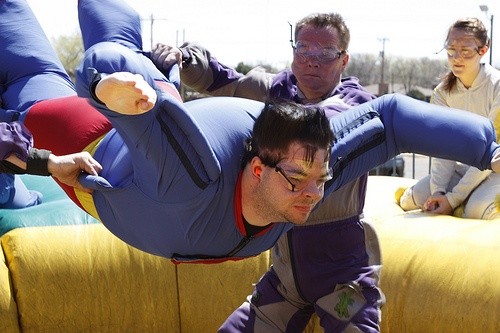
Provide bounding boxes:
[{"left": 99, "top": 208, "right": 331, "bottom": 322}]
[
  {"left": 260, "top": 158, "right": 333, "bottom": 193},
  {"left": 293, "top": 41, "right": 347, "bottom": 63},
  {"left": 444, "top": 38, "right": 483, "bottom": 59}
]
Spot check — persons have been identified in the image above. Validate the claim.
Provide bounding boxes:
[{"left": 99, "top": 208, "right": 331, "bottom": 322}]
[
  {"left": 0, "top": 120, "right": 103, "bottom": 195},
  {"left": 150, "top": 13, "right": 385, "bottom": 333},
  {"left": 399, "top": 18, "right": 500, "bottom": 220},
  {"left": 0, "top": 0, "right": 500, "bottom": 265}
]
